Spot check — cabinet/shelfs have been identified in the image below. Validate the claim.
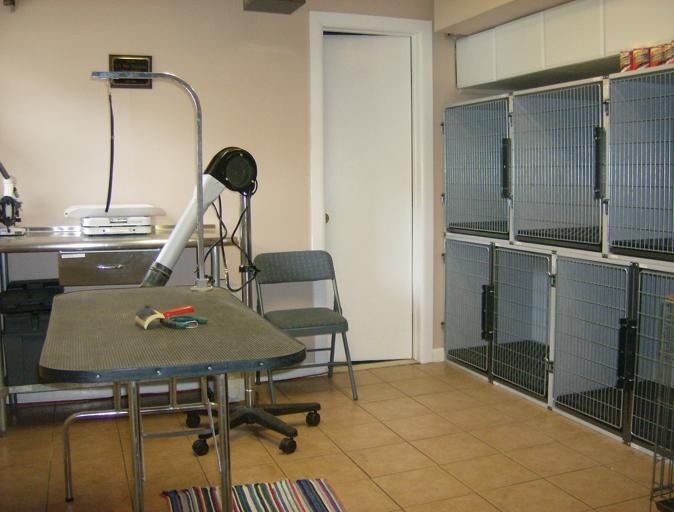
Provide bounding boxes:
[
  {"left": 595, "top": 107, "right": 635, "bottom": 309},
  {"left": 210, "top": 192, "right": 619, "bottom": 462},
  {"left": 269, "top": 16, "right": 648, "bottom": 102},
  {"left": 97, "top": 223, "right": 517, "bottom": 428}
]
[
  {"left": 443, "top": 95, "right": 510, "bottom": 241},
  {"left": 604, "top": 66, "right": 674, "bottom": 263},
  {"left": 1, "top": 223, "right": 239, "bottom": 437},
  {"left": 446, "top": 238, "right": 550, "bottom": 409},
  {"left": 551, "top": 250, "right": 674, "bottom": 460},
  {"left": 510, "top": 74, "right": 604, "bottom": 254}
]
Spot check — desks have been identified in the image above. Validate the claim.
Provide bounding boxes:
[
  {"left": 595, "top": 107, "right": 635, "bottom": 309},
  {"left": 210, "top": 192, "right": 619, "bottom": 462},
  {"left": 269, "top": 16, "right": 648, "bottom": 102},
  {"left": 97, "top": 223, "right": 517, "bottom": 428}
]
[{"left": 39, "top": 284, "right": 308, "bottom": 512}]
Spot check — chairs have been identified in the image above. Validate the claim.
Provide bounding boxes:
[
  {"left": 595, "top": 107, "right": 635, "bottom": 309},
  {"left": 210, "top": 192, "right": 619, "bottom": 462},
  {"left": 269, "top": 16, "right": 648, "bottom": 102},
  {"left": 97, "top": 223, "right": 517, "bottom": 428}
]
[{"left": 249, "top": 250, "right": 360, "bottom": 402}]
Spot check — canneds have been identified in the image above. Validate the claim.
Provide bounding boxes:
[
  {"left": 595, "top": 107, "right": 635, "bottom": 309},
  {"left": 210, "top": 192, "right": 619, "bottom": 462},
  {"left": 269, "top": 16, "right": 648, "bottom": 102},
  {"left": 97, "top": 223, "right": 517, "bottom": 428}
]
[{"left": 619, "top": 41, "right": 674, "bottom": 73}]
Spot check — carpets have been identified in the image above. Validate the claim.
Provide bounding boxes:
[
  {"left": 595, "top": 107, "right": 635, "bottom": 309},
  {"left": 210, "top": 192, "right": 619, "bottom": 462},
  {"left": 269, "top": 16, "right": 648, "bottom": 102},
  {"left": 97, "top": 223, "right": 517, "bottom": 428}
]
[{"left": 160, "top": 475, "right": 347, "bottom": 512}]
